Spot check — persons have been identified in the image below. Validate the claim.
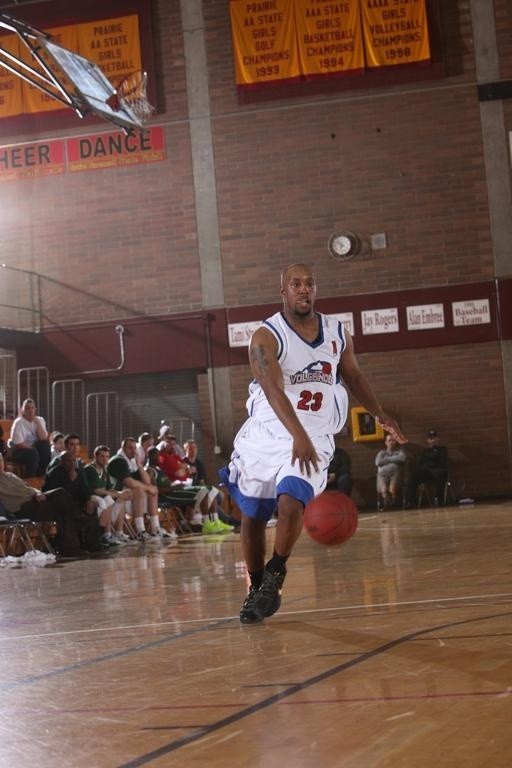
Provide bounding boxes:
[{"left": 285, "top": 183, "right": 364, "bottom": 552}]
[
  {"left": 374, "top": 434, "right": 406, "bottom": 511},
  {"left": 324, "top": 447, "right": 354, "bottom": 496},
  {"left": 218, "top": 263, "right": 409, "bottom": 623},
  {"left": 0, "top": 396, "right": 235, "bottom": 559},
  {"left": 416, "top": 430, "right": 449, "bottom": 508},
  {"left": 359, "top": 414, "right": 375, "bottom": 436}
]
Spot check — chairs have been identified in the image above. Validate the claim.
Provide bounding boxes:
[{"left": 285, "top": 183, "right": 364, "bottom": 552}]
[
  {"left": 349, "top": 462, "right": 459, "bottom": 514},
  {"left": 0, "top": 413, "right": 193, "bottom": 561}
]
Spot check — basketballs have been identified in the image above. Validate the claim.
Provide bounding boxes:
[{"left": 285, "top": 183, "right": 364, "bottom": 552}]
[{"left": 303, "top": 492, "right": 358, "bottom": 544}]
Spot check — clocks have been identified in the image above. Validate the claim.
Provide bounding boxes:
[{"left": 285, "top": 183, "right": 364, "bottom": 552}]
[{"left": 331, "top": 236, "right": 352, "bottom": 256}]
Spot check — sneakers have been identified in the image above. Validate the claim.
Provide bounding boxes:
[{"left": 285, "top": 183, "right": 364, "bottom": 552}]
[
  {"left": 254, "top": 562, "right": 286, "bottom": 617},
  {"left": 240, "top": 584, "right": 264, "bottom": 623},
  {"left": 151, "top": 527, "right": 177, "bottom": 539},
  {"left": 228, "top": 517, "right": 241, "bottom": 527},
  {"left": 202, "top": 520, "right": 224, "bottom": 533},
  {"left": 214, "top": 519, "right": 234, "bottom": 530},
  {"left": 136, "top": 529, "right": 158, "bottom": 542},
  {"left": 116, "top": 533, "right": 138, "bottom": 545},
  {"left": 102, "top": 535, "right": 128, "bottom": 549}
]
